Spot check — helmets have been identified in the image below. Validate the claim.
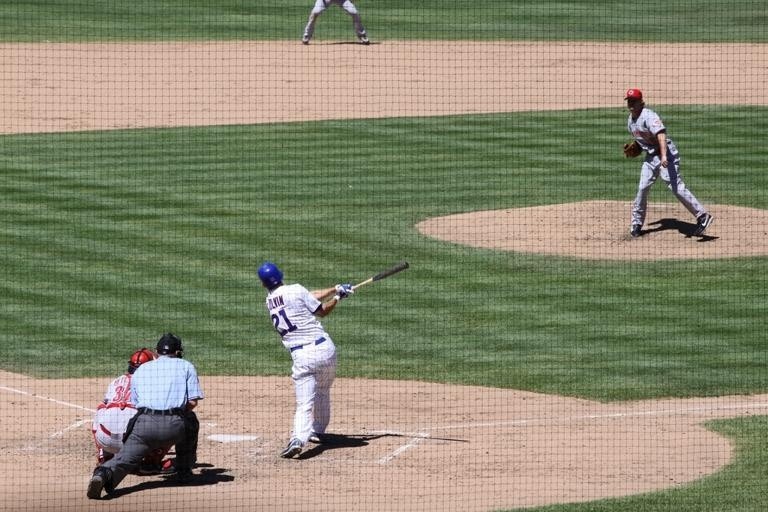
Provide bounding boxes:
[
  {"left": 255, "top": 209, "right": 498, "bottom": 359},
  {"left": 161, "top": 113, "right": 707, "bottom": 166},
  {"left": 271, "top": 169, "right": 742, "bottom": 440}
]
[
  {"left": 126, "top": 347, "right": 156, "bottom": 368},
  {"left": 155, "top": 332, "right": 182, "bottom": 356},
  {"left": 624, "top": 89, "right": 642, "bottom": 100},
  {"left": 258, "top": 262, "right": 283, "bottom": 287}
]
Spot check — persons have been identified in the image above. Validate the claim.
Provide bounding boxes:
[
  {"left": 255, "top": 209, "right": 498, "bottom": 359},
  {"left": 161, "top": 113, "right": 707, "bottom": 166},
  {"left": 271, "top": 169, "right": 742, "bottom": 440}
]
[
  {"left": 92, "top": 348, "right": 175, "bottom": 474},
  {"left": 258, "top": 263, "right": 355, "bottom": 460},
  {"left": 624, "top": 89, "right": 714, "bottom": 236},
  {"left": 87, "top": 333, "right": 204, "bottom": 500},
  {"left": 302, "top": 0, "right": 369, "bottom": 45}
]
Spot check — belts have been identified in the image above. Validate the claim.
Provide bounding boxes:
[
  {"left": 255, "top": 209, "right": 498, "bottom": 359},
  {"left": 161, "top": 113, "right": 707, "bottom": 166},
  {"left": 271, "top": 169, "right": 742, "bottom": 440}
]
[
  {"left": 140, "top": 407, "right": 179, "bottom": 415},
  {"left": 290, "top": 336, "right": 326, "bottom": 353}
]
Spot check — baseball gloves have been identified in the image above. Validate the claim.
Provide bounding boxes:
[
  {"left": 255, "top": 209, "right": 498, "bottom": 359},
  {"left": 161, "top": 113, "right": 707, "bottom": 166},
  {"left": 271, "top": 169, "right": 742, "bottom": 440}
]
[{"left": 624, "top": 141, "right": 642, "bottom": 158}]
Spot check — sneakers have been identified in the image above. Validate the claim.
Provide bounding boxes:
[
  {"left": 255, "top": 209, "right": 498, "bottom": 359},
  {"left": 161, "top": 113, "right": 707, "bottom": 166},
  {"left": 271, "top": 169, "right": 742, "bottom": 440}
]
[
  {"left": 695, "top": 213, "right": 714, "bottom": 235},
  {"left": 630, "top": 223, "right": 642, "bottom": 236},
  {"left": 138, "top": 459, "right": 175, "bottom": 474},
  {"left": 171, "top": 469, "right": 195, "bottom": 485},
  {"left": 86, "top": 468, "right": 108, "bottom": 498},
  {"left": 310, "top": 431, "right": 323, "bottom": 443},
  {"left": 280, "top": 437, "right": 302, "bottom": 458}
]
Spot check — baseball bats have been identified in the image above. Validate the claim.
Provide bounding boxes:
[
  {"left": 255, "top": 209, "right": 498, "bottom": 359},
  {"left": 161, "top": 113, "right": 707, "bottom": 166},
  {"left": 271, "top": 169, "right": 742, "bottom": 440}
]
[{"left": 351, "top": 262, "right": 408, "bottom": 290}]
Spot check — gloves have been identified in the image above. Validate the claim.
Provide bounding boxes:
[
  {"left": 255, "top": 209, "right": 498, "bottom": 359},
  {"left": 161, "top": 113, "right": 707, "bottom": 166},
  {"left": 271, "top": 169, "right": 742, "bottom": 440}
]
[{"left": 334, "top": 284, "right": 351, "bottom": 300}]
